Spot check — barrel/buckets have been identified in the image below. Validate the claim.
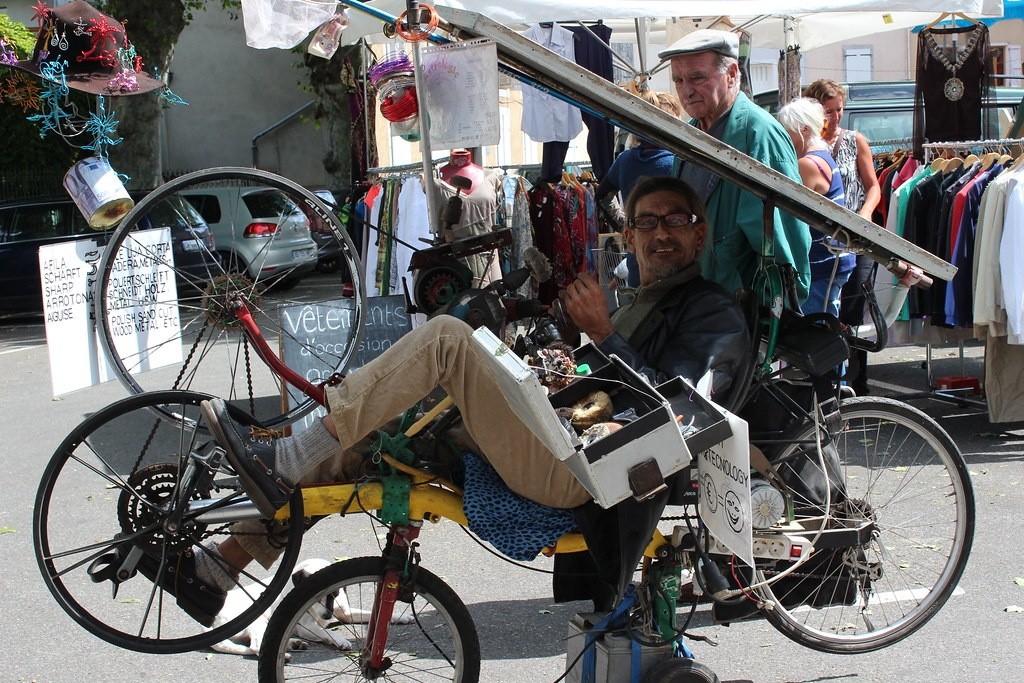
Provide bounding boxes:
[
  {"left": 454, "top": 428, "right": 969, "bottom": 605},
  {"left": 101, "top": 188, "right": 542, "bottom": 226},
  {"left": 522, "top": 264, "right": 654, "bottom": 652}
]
[{"left": 62, "top": 157, "right": 135, "bottom": 229}]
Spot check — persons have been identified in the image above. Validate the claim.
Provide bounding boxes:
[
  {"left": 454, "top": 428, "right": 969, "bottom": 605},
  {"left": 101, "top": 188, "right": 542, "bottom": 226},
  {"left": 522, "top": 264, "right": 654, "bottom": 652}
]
[
  {"left": 427, "top": 148, "right": 503, "bottom": 290},
  {"left": 656, "top": 29, "right": 812, "bottom": 375},
  {"left": 595, "top": 92, "right": 684, "bottom": 292},
  {"left": 775, "top": 95, "right": 858, "bottom": 404},
  {"left": 112, "top": 173, "right": 757, "bottom": 628},
  {"left": 797, "top": 78, "right": 881, "bottom": 397}
]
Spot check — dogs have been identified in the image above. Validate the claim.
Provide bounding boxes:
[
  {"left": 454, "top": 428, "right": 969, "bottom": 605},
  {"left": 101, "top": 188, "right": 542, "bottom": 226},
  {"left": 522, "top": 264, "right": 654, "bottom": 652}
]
[{"left": 202, "top": 558, "right": 416, "bottom": 662}]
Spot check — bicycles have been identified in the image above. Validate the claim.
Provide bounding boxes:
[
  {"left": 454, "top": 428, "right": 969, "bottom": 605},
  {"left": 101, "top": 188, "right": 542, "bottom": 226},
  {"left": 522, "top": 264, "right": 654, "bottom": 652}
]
[{"left": 31, "top": 0, "right": 975, "bottom": 683}]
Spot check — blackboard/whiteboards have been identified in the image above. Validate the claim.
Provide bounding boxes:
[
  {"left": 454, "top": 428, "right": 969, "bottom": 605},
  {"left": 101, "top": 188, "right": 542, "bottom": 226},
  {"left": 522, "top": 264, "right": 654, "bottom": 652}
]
[{"left": 276, "top": 294, "right": 413, "bottom": 439}]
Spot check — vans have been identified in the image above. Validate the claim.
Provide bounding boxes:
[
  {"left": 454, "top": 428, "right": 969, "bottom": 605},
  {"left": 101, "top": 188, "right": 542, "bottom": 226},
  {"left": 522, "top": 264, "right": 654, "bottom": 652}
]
[{"left": 753, "top": 74, "right": 1024, "bottom": 173}]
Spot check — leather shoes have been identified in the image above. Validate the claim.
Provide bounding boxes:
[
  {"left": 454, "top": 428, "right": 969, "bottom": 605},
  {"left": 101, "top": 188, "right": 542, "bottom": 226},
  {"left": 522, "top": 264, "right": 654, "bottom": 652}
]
[
  {"left": 113, "top": 532, "right": 227, "bottom": 628},
  {"left": 200, "top": 395, "right": 297, "bottom": 518}
]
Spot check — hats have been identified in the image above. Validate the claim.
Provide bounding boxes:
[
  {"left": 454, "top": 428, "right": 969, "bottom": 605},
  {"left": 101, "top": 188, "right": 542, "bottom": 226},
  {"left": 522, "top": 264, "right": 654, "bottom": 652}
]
[
  {"left": 657, "top": 28, "right": 739, "bottom": 63},
  {"left": 0, "top": 0, "right": 166, "bottom": 96}
]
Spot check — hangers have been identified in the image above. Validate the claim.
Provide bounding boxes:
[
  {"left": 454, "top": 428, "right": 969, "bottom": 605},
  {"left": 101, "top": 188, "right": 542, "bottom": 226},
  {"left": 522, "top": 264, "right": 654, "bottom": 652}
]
[
  {"left": 868, "top": 137, "right": 1024, "bottom": 197},
  {"left": 368, "top": 158, "right": 601, "bottom": 206},
  {"left": 920, "top": 12, "right": 988, "bottom": 33}
]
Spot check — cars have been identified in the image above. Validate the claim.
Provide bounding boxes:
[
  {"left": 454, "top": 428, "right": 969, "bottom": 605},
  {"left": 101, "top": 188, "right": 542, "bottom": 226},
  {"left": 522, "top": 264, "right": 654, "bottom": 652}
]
[
  {"left": 288, "top": 191, "right": 344, "bottom": 261},
  {"left": 0, "top": 189, "right": 223, "bottom": 318},
  {"left": 176, "top": 187, "right": 318, "bottom": 296}
]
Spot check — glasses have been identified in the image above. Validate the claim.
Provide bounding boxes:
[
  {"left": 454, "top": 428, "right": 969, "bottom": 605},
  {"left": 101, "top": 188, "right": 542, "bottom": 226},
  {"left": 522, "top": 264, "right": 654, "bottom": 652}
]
[
  {"left": 825, "top": 106, "right": 843, "bottom": 114},
  {"left": 628, "top": 211, "right": 706, "bottom": 229}
]
